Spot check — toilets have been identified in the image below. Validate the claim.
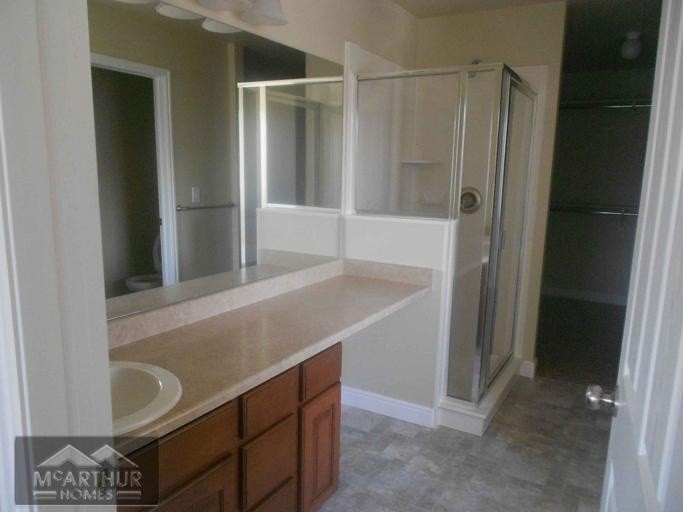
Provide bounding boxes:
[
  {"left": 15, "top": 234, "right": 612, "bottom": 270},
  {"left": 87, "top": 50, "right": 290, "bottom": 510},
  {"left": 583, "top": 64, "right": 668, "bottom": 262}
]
[{"left": 126, "top": 234, "right": 161, "bottom": 292}]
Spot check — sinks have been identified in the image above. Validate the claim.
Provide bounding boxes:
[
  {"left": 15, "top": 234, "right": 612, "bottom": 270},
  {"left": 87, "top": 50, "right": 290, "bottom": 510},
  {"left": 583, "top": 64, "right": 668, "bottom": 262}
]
[{"left": 110, "top": 359, "right": 183, "bottom": 437}]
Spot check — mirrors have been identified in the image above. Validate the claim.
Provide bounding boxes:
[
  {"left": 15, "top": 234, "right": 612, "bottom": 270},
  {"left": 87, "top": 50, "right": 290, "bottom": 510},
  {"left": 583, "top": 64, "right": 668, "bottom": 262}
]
[{"left": 88, "top": 3, "right": 347, "bottom": 350}]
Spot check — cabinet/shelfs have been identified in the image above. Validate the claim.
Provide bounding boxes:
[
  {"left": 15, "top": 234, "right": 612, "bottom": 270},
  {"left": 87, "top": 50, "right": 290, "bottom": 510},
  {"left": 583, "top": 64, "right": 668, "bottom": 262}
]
[{"left": 114, "top": 341, "right": 340, "bottom": 511}]
